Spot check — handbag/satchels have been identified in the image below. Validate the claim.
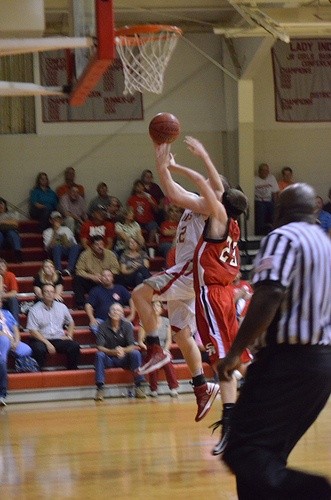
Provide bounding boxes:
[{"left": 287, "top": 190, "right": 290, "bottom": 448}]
[{"left": 15, "top": 356, "right": 40, "bottom": 372}]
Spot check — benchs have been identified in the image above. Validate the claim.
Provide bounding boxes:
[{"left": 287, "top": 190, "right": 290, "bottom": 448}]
[{"left": 1, "top": 221, "right": 215, "bottom": 391}]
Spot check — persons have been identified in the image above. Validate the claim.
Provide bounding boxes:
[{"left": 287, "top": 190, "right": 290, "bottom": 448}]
[
  {"left": 216, "top": 182, "right": 331, "bottom": 500},
  {"left": 232, "top": 270, "right": 256, "bottom": 394},
  {"left": 254, "top": 163, "right": 281, "bottom": 234},
  {"left": 132, "top": 140, "right": 232, "bottom": 422},
  {"left": 0, "top": 308, "right": 20, "bottom": 407},
  {"left": 315, "top": 196, "right": 331, "bottom": 235},
  {"left": 0, "top": 166, "right": 201, "bottom": 401},
  {"left": 168, "top": 135, "right": 249, "bottom": 456},
  {"left": 277, "top": 166, "right": 297, "bottom": 190}
]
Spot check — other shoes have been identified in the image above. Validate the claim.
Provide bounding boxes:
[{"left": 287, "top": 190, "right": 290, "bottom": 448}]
[
  {"left": 56, "top": 269, "right": 62, "bottom": 274},
  {"left": 63, "top": 269, "right": 70, "bottom": 276},
  {"left": 170, "top": 390, "right": 178, "bottom": 397},
  {"left": 150, "top": 391, "right": 158, "bottom": 397}
]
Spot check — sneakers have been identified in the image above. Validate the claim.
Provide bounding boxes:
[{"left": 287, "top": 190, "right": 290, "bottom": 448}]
[
  {"left": 94, "top": 391, "right": 103, "bottom": 400},
  {"left": 136, "top": 388, "right": 147, "bottom": 398},
  {"left": 136, "top": 344, "right": 169, "bottom": 375},
  {"left": 210, "top": 417, "right": 231, "bottom": 455},
  {"left": 192, "top": 381, "right": 220, "bottom": 423}
]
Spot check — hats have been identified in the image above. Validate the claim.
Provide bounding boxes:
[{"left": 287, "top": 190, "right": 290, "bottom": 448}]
[{"left": 50, "top": 211, "right": 61, "bottom": 219}]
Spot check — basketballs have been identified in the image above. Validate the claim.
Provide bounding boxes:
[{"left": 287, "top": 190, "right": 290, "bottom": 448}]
[{"left": 149, "top": 112, "right": 180, "bottom": 145}]
